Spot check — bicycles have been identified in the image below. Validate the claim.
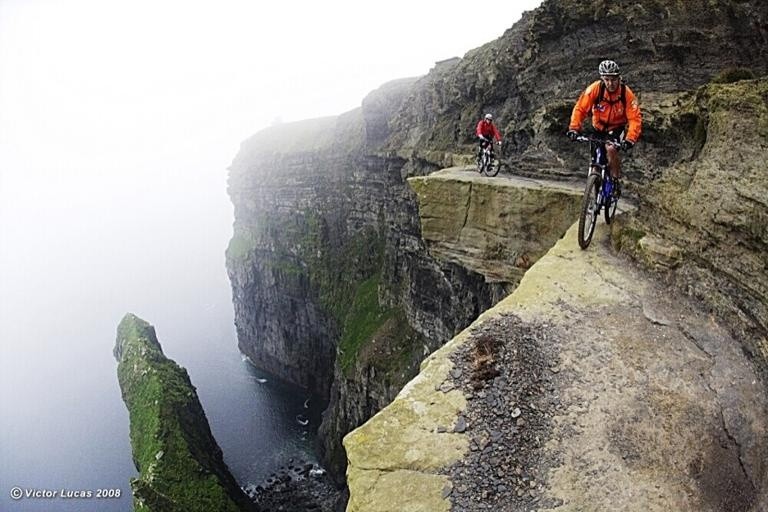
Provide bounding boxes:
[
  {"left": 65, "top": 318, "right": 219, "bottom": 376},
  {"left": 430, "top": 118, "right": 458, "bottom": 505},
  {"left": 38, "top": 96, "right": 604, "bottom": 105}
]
[
  {"left": 476, "top": 137, "right": 500, "bottom": 177},
  {"left": 567, "top": 132, "right": 623, "bottom": 249}
]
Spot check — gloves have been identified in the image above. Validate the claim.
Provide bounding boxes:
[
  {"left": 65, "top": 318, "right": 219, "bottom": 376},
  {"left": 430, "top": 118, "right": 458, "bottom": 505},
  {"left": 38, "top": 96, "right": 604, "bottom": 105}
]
[
  {"left": 566, "top": 129, "right": 579, "bottom": 142},
  {"left": 620, "top": 140, "right": 633, "bottom": 152}
]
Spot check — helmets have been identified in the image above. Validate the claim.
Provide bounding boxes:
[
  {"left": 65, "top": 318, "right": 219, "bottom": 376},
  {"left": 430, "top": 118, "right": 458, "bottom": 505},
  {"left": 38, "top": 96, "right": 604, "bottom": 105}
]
[
  {"left": 485, "top": 114, "right": 493, "bottom": 120},
  {"left": 598, "top": 60, "right": 621, "bottom": 76}
]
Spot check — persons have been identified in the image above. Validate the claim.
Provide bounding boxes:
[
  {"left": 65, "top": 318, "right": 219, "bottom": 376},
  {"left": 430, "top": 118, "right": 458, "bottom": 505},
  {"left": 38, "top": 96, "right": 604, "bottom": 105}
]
[
  {"left": 566, "top": 58, "right": 644, "bottom": 213},
  {"left": 474, "top": 113, "right": 503, "bottom": 165}
]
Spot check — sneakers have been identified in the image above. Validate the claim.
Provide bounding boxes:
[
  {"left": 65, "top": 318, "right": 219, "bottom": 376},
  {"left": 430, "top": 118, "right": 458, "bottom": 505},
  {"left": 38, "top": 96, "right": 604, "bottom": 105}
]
[{"left": 609, "top": 176, "right": 621, "bottom": 198}]
